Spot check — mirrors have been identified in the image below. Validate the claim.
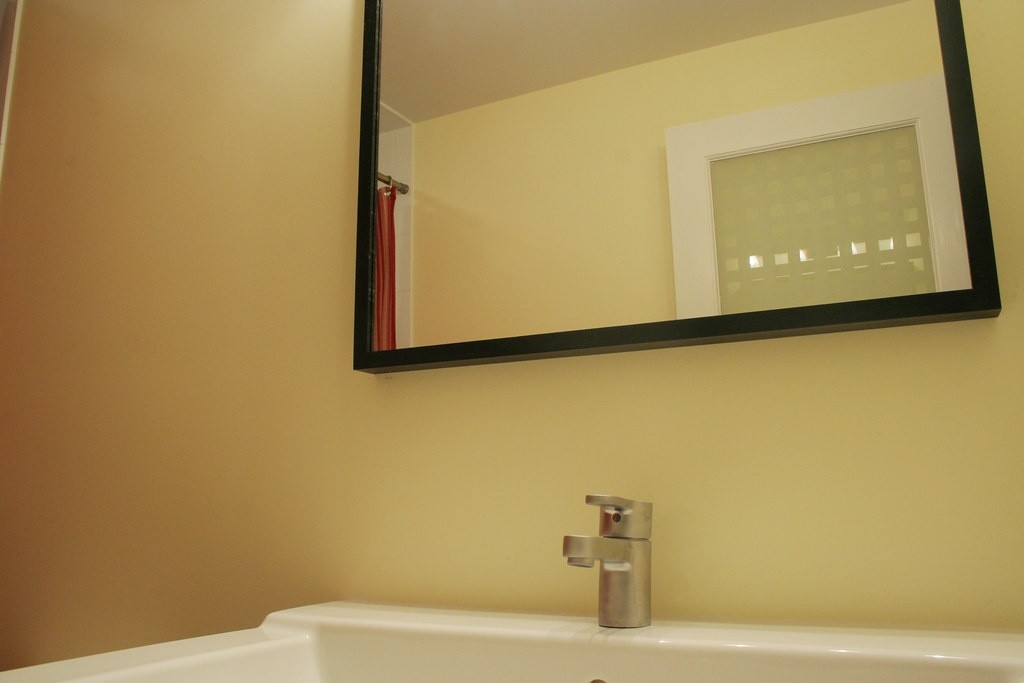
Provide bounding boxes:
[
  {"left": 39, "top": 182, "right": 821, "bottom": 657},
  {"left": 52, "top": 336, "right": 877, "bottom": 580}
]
[{"left": 352, "top": 0, "right": 1002, "bottom": 376}]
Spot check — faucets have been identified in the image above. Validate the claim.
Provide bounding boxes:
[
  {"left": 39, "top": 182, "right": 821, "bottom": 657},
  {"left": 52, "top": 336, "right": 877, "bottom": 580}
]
[{"left": 561, "top": 492, "right": 654, "bottom": 631}]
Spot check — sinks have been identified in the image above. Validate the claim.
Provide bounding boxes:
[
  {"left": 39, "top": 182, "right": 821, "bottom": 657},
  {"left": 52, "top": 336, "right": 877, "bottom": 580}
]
[{"left": 0, "top": 599, "right": 1024, "bottom": 683}]
